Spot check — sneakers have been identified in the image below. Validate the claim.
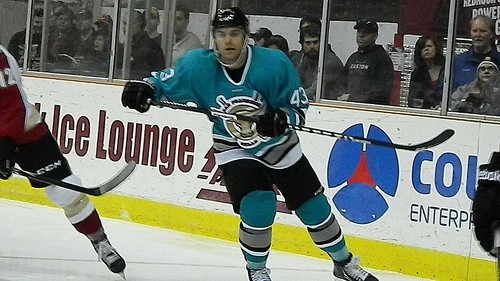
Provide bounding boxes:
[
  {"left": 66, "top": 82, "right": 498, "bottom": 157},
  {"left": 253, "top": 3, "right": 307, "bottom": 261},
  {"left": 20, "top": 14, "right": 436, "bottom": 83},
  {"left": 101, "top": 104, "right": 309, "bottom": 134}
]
[
  {"left": 245, "top": 265, "right": 272, "bottom": 281},
  {"left": 90, "top": 233, "right": 127, "bottom": 281},
  {"left": 326, "top": 251, "right": 379, "bottom": 281}
]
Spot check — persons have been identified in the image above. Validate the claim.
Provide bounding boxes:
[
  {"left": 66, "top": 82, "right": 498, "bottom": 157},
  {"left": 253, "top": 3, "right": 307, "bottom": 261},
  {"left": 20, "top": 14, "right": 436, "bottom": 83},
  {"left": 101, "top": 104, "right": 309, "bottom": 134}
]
[
  {"left": 408, "top": 36, "right": 445, "bottom": 108},
  {"left": 249, "top": 17, "right": 344, "bottom": 98},
  {"left": 437, "top": 15, "right": 500, "bottom": 99},
  {"left": 8, "top": 0, "right": 202, "bottom": 79},
  {"left": 0, "top": 45, "right": 126, "bottom": 279},
  {"left": 472, "top": 151, "right": 500, "bottom": 281},
  {"left": 121, "top": 8, "right": 379, "bottom": 280},
  {"left": 334, "top": 18, "right": 394, "bottom": 105},
  {"left": 447, "top": 57, "right": 500, "bottom": 116}
]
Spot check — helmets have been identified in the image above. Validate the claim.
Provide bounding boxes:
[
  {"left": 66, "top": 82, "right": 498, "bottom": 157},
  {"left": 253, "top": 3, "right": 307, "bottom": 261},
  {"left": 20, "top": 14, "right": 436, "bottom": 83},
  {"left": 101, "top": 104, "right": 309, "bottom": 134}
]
[{"left": 210, "top": 6, "right": 250, "bottom": 38}]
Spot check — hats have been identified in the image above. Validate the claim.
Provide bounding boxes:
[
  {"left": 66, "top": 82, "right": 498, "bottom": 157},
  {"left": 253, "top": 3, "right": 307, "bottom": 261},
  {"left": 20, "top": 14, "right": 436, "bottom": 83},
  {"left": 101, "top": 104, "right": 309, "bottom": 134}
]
[
  {"left": 264, "top": 35, "right": 289, "bottom": 54},
  {"left": 353, "top": 17, "right": 378, "bottom": 35},
  {"left": 476, "top": 52, "right": 500, "bottom": 75},
  {"left": 73, "top": 8, "right": 94, "bottom": 19},
  {"left": 143, "top": 7, "right": 160, "bottom": 20},
  {"left": 249, "top": 27, "right": 272, "bottom": 40},
  {"left": 95, "top": 14, "right": 113, "bottom": 28}
]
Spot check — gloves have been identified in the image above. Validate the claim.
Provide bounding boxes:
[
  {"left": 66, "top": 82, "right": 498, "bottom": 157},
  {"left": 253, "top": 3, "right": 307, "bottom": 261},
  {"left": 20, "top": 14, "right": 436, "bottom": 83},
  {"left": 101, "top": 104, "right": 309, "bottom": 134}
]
[
  {"left": 256, "top": 106, "right": 288, "bottom": 139},
  {"left": 0, "top": 135, "right": 20, "bottom": 181},
  {"left": 121, "top": 78, "right": 156, "bottom": 114}
]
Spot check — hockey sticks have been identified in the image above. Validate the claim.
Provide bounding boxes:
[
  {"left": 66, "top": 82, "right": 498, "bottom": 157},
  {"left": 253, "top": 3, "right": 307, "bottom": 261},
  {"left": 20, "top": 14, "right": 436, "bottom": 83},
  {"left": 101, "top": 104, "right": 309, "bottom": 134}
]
[
  {"left": 0, "top": 160, "right": 137, "bottom": 196},
  {"left": 147, "top": 98, "right": 455, "bottom": 152}
]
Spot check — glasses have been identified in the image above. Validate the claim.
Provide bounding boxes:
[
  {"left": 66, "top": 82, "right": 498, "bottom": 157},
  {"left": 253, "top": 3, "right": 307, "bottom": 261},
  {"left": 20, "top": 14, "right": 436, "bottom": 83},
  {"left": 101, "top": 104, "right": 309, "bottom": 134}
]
[
  {"left": 57, "top": 16, "right": 70, "bottom": 23},
  {"left": 98, "top": 22, "right": 108, "bottom": 29},
  {"left": 356, "top": 19, "right": 373, "bottom": 26},
  {"left": 304, "top": 39, "right": 319, "bottom": 45},
  {"left": 479, "top": 66, "right": 496, "bottom": 72}
]
